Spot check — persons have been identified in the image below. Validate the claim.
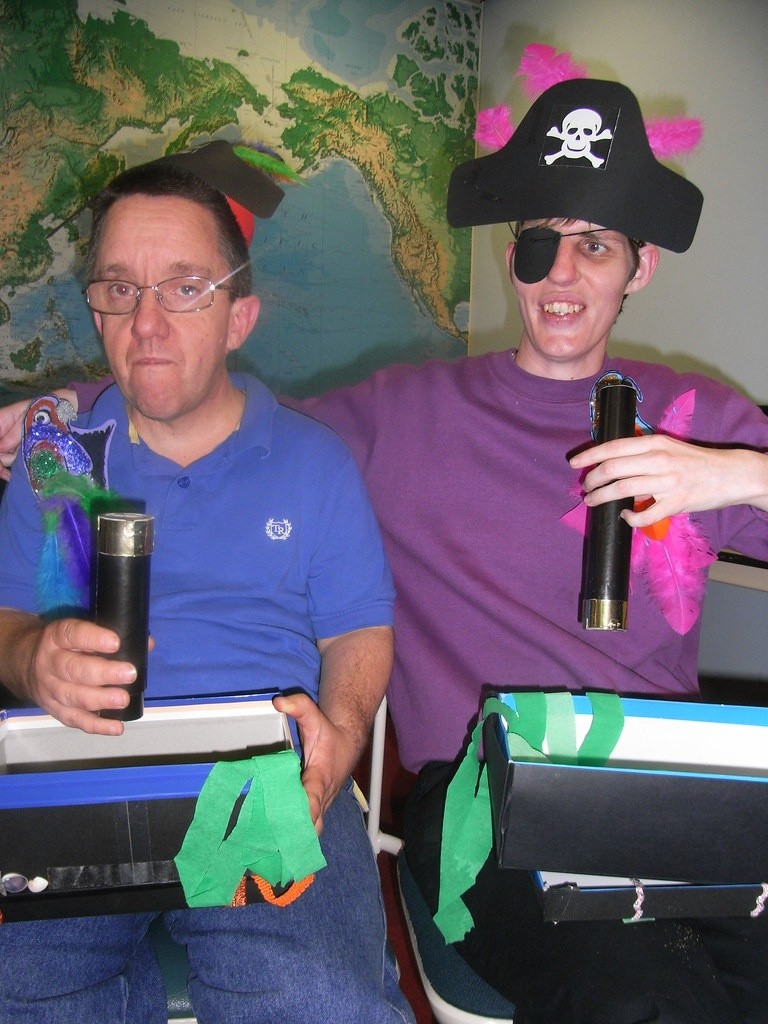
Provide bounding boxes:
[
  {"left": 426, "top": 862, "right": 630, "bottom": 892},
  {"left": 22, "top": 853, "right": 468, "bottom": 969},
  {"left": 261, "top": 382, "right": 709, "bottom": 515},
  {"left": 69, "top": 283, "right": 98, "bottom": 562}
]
[
  {"left": 1, "top": 79, "right": 767, "bottom": 1023},
  {"left": 2, "top": 164, "right": 418, "bottom": 1023}
]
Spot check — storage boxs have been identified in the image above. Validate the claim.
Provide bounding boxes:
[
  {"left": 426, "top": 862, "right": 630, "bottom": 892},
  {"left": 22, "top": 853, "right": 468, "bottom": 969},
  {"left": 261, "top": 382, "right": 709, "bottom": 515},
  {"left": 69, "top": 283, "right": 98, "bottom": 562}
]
[
  {"left": 1, "top": 694, "right": 304, "bottom": 923},
  {"left": 481, "top": 686, "right": 768, "bottom": 923}
]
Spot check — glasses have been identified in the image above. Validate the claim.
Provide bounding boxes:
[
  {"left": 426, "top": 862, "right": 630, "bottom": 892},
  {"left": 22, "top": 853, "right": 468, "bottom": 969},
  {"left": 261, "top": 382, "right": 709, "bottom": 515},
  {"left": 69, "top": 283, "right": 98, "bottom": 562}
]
[{"left": 81, "top": 275, "right": 243, "bottom": 315}]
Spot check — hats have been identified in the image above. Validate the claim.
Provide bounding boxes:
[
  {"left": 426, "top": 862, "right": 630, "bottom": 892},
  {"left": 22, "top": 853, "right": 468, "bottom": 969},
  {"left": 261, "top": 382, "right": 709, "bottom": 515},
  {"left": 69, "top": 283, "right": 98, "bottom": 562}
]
[{"left": 447, "top": 44, "right": 704, "bottom": 254}]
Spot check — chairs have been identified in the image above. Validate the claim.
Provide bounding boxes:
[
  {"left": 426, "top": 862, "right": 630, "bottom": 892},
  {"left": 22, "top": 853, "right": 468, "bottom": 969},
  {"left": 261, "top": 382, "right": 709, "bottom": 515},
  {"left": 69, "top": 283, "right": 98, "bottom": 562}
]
[{"left": 142, "top": 691, "right": 517, "bottom": 1024}]
